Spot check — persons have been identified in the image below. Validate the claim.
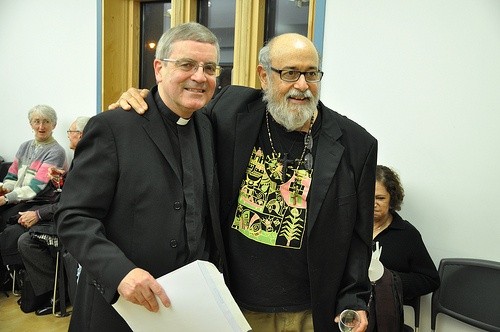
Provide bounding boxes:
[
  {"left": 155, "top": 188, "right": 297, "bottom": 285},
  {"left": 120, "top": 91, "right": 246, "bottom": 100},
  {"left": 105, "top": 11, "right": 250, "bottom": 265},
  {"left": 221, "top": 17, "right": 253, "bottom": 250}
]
[
  {"left": 0, "top": 105, "right": 92, "bottom": 317},
  {"left": 54, "top": 22, "right": 225, "bottom": 331},
  {"left": 106, "top": 32, "right": 378, "bottom": 331},
  {"left": 368, "top": 164, "right": 441, "bottom": 332}
]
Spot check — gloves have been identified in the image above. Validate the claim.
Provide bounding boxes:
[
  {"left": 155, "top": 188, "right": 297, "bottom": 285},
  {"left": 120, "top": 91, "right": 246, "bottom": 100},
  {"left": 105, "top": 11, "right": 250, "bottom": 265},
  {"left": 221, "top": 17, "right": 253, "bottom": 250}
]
[{"left": 367, "top": 241, "right": 385, "bottom": 281}]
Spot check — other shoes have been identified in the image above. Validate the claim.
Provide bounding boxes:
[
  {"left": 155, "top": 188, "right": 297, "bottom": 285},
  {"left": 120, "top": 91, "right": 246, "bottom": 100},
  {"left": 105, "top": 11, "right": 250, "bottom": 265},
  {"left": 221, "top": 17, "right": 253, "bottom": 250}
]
[{"left": 36, "top": 297, "right": 60, "bottom": 315}]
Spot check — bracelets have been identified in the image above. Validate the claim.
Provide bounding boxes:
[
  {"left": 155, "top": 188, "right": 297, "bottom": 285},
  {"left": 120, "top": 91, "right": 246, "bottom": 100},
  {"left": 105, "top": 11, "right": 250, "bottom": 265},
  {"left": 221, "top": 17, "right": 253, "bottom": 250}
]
[{"left": 3, "top": 195, "right": 8, "bottom": 204}]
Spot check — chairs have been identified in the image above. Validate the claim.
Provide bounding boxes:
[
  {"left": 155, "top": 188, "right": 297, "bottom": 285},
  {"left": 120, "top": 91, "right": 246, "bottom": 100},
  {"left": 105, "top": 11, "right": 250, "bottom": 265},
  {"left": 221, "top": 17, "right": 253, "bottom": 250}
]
[{"left": 403, "top": 258, "right": 500, "bottom": 332}]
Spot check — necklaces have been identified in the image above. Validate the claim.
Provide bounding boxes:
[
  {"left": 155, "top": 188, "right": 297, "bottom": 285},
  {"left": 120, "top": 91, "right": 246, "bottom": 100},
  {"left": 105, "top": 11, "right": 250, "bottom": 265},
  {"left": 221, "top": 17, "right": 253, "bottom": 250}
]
[
  {"left": 265, "top": 108, "right": 314, "bottom": 183},
  {"left": 373, "top": 214, "right": 390, "bottom": 233}
]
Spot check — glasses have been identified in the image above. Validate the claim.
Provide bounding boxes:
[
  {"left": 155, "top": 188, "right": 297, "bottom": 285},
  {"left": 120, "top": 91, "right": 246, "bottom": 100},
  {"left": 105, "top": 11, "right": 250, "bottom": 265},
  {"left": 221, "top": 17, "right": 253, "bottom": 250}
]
[
  {"left": 67, "top": 130, "right": 81, "bottom": 136},
  {"left": 162, "top": 58, "right": 221, "bottom": 77},
  {"left": 270, "top": 67, "right": 323, "bottom": 83}
]
